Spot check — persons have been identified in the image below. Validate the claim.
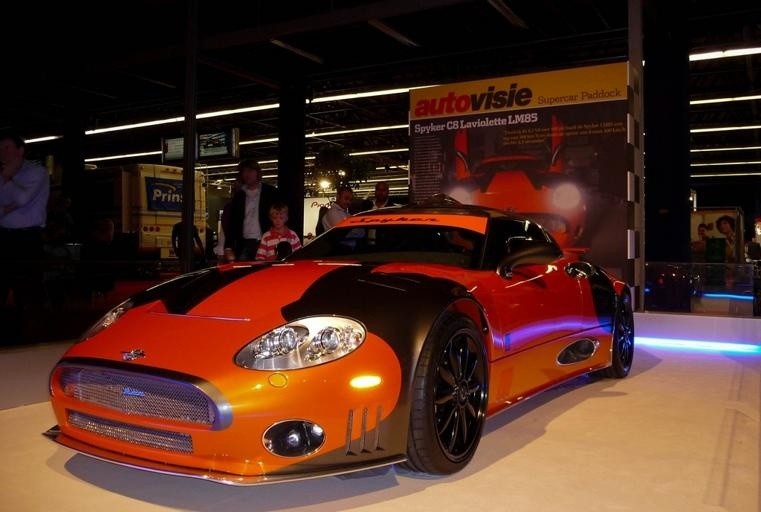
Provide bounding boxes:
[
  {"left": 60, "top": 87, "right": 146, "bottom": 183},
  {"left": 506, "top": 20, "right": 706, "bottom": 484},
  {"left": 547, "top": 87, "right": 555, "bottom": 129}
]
[
  {"left": 172, "top": 159, "right": 302, "bottom": 276},
  {"left": 0, "top": 136, "right": 50, "bottom": 348},
  {"left": 317, "top": 182, "right": 397, "bottom": 236},
  {"left": 689, "top": 215, "right": 737, "bottom": 257},
  {"left": 80, "top": 216, "right": 125, "bottom": 279}
]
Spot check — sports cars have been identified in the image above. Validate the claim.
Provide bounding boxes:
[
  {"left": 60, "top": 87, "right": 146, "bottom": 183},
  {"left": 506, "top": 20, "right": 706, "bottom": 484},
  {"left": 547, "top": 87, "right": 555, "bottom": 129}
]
[
  {"left": 439, "top": 115, "right": 593, "bottom": 249},
  {"left": 41, "top": 196, "right": 643, "bottom": 484}
]
